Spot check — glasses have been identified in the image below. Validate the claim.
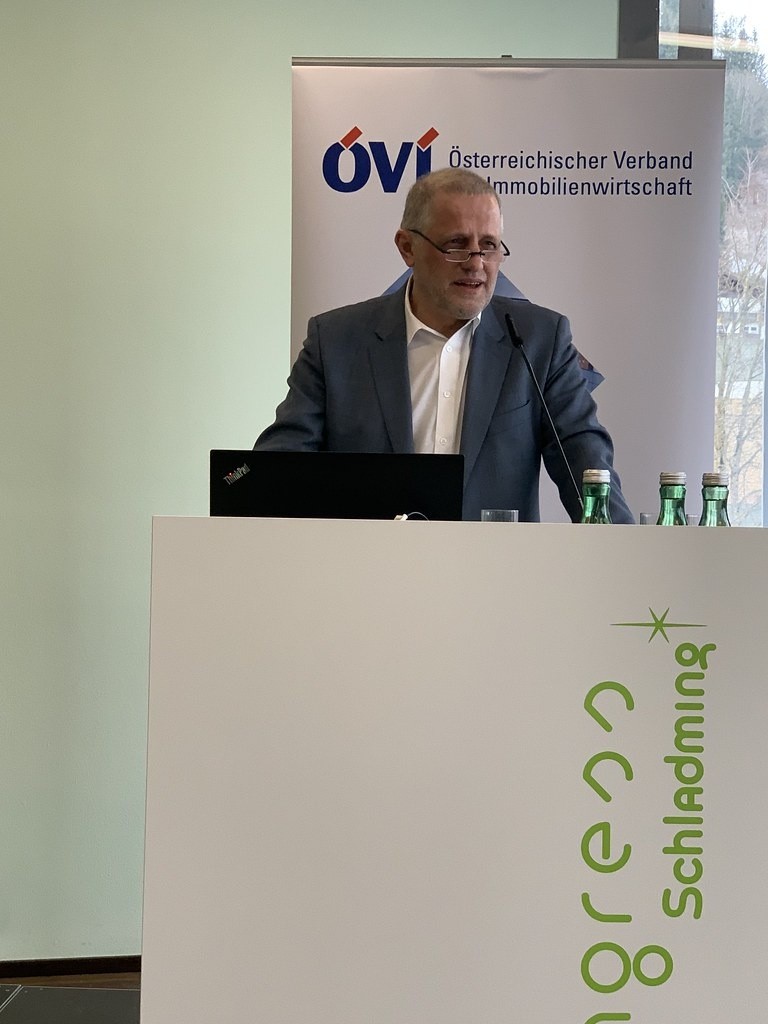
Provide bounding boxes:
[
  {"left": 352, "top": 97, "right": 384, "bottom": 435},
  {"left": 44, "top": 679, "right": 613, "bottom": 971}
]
[{"left": 407, "top": 228, "right": 511, "bottom": 263}]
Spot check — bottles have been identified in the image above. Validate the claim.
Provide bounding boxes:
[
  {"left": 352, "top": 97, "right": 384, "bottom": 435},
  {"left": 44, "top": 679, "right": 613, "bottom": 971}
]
[
  {"left": 579, "top": 469, "right": 612, "bottom": 524},
  {"left": 655, "top": 472, "right": 688, "bottom": 526},
  {"left": 697, "top": 472, "right": 732, "bottom": 526}
]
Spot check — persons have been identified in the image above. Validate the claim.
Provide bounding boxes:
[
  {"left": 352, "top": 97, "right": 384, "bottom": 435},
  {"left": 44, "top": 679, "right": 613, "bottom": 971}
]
[{"left": 253, "top": 168, "right": 638, "bottom": 524}]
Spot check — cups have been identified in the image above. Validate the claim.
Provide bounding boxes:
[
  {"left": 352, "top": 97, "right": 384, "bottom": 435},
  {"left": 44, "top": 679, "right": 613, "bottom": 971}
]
[
  {"left": 639, "top": 512, "right": 702, "bottom": 526},
  {"left": 480, "top": 509, "right": 519, "bottom": 522}
]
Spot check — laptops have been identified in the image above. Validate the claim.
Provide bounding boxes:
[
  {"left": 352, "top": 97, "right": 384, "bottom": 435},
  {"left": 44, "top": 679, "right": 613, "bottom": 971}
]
[{"left": 209, "top": 449, "right": 465, "bottom": 522}]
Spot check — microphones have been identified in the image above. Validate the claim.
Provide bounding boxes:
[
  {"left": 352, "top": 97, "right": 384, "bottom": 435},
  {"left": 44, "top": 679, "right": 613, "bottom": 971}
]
[{"left": 504, "top": 313, "right": 585, "bottom": 513}]
